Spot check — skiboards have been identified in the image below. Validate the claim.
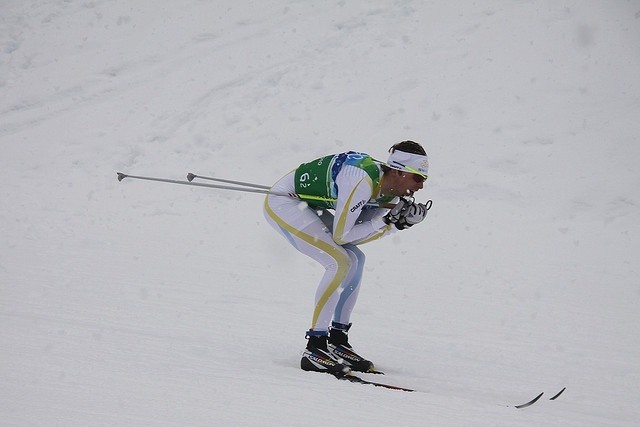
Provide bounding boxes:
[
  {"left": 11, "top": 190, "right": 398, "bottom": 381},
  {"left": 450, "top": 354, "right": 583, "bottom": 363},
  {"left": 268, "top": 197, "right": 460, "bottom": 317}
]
[{"left": 345, "top": 369, "right": 566, "bottom": 408}]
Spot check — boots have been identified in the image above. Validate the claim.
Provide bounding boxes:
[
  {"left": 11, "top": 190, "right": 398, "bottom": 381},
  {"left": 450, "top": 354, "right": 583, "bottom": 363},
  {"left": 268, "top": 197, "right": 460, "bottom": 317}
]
[
  {"left": 328, "top": 320, "right": 375, "bottom": 372},
  {"left": 299, "top": 329, "right": 356, "bottom": 374}
]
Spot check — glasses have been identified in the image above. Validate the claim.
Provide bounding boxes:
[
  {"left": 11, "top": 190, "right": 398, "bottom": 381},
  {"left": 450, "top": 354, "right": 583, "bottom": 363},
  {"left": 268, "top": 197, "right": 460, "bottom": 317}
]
[{"left": 409, "top": 172, "right": 425, "bottom": 184}]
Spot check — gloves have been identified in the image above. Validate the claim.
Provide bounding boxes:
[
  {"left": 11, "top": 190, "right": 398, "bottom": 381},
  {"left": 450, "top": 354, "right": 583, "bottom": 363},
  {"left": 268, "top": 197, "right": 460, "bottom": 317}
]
[
  {"left": 394, "top": 200, "right": 433, "bottom": 231},
  {"left": 382, "top": 198, "right": 415, "bottom": 226}
]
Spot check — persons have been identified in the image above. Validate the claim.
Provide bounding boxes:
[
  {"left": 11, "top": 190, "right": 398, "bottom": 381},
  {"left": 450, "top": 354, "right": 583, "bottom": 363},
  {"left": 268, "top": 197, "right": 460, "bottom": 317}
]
[{"left": 263, "top": 140, "right": 428, "bottom": 379}]
